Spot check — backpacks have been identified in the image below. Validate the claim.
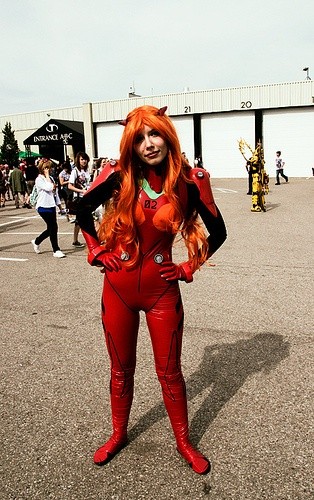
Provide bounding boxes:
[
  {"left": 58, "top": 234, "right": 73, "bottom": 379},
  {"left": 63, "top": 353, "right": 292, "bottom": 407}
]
[{"left": 30, "top": 177, "right": 56, "bottom": 206}]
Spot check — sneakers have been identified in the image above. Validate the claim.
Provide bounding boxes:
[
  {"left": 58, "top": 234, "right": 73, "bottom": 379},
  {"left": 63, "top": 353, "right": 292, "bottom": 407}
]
[
  {"left": 72, "top": 241, "right": 85, "bottom": 247},
  {"left": 30, "top": 239, "right": 40, "bottom": 253},
  {"left": 52, "top": 250, "right": 65, "bottom": 257}
]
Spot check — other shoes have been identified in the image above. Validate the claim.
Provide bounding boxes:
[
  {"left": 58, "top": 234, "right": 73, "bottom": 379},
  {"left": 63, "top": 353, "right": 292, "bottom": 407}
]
[
  {"left": 15, "top": 204, "right": 19, "bottom": 208},
  {"left": 247, "top": 193, "right": 252, "bottom": 195},
  {"left": 275, "top": 183, "right": 280, "bottom": 185},
  {"left": 25, "top": 203, "right": 31, "bottom": 209},
  {"left": 286, "top": 177, "right": 288, "bottom": 182}
]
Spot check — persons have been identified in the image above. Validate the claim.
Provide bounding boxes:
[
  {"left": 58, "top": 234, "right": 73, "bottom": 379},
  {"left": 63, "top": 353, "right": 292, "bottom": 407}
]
[
  {"left": 180, "top": 151, "right": 289, "bottom": 196},
  {"left": 237, "top": 136, "right": 270, "bottom": 212},
  {"left": 0, "top": 152, "right": 113, "bottom": 258},
  {"left": 75, "top": 105, "right": 227, "bottom": 474}
]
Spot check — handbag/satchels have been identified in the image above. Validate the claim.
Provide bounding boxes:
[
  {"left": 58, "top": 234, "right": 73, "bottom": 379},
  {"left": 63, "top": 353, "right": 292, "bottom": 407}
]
[{"left": 68, "top": 198, "right": 79, "bottom": 215}]
